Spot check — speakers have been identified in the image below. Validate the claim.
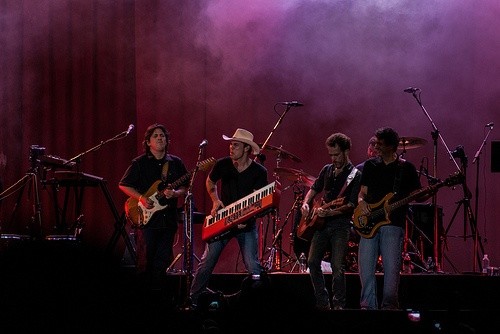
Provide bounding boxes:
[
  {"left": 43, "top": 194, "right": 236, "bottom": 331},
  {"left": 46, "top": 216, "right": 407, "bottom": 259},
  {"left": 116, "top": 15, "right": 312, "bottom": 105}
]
[{"left": 408, "top": 203, "right": 443, "bottom": 263}]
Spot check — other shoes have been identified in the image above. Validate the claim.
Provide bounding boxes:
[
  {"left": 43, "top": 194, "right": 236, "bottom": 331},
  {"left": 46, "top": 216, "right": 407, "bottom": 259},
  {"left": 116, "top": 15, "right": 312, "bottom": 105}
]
[
  {"left": 176, "top": 302, "right": 194, "bottom": 311},
  {"left": 317, "top": 302, "right": 330, "bottom": 308},
  {"left": 361, "top": 306, "right": 367, "bottom": 310}
]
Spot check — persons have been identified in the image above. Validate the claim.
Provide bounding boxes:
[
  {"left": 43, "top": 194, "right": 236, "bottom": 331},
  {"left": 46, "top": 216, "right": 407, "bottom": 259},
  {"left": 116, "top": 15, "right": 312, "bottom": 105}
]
[
  {"left": 118, "top": 123, "right": 188, "bottom": 276},
  {"left": 300, "top": 133, "right": 362, "bottom": 311},
  {"left": 355, "top": 136, "right": 383, "bottom": 172},
  {"left": 357, "top": 127, "right": 439, "bottom": 312},
  {"left": 178, "top": 128, "right": 269, "bottom": 312}
]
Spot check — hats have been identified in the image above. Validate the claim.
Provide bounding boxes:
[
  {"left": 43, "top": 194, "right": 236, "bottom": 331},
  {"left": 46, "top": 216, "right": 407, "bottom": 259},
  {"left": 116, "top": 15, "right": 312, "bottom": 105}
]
[
  {"left": 368, "top": 136, "right": 377, "bottom": 144},
  {"left": 222, "top": 128, "right": 260, "bottom": 155}
]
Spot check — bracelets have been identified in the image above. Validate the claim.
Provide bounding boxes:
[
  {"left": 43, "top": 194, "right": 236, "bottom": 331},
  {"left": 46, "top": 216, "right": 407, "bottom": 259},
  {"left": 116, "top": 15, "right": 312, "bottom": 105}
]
[{"left": 138, "top": 195, "right": 143, "bottom": 202}]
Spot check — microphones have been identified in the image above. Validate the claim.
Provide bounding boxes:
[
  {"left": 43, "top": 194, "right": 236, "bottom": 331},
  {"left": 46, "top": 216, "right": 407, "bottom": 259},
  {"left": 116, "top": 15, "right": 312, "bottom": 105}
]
[
  {"left": 126, "top": 124, "right": 134, "bottom": 136},
  {"left": 283, "top": 102, "right": 304, "bottom": 106},
  {"left": 485, "top": 122, "right": 494, "bottom": 127},
  {"left": 199, "top": 139, "right": 208, "bottom": 148},
  {"left": 403, "top": 88, "right": 419, "bottom": 92}
]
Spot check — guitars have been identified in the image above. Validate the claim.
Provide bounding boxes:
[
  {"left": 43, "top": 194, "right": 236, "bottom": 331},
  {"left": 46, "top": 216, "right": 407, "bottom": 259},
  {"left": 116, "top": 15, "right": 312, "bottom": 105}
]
[
  {"left": 124, "top": 156, "right": 216, "bottom": 229},
  {"left": 352, "top": 169, "right": 464, "bottom": 239}
]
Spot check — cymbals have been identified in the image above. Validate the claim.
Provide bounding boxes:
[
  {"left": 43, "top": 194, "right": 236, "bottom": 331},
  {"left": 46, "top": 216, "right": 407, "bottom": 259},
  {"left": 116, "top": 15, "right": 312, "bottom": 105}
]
[
  {"left": 256, "top": 141, "right": 304, "bottom": 164},
  {"left": 394, "top": 136, "right": 429, "bottom": 149},
  {"left": 274, "top": 165, "right": 316, "bottom": 187}
]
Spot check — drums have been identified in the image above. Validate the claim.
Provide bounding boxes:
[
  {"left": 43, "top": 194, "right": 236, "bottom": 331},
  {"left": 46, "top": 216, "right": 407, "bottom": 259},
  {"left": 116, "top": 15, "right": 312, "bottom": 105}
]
[
  {"left": 407, "top": 203, "right": 444, "bottom": 240},
  {"left": 296, "top": 195, "right": 348, "bottom": 241}
]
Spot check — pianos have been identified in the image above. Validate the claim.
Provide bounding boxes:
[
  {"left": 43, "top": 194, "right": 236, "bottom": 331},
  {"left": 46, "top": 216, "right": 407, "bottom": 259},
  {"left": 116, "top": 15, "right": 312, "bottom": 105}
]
[{"left": 201, "top": 180, "right": 281, "bottom": 245}]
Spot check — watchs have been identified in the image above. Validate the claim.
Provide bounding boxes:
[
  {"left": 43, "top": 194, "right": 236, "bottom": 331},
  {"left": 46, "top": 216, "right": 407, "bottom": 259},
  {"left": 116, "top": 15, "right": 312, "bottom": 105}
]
[{"left": 359, "top": 198, "right": 363, "bottom": 201}]
